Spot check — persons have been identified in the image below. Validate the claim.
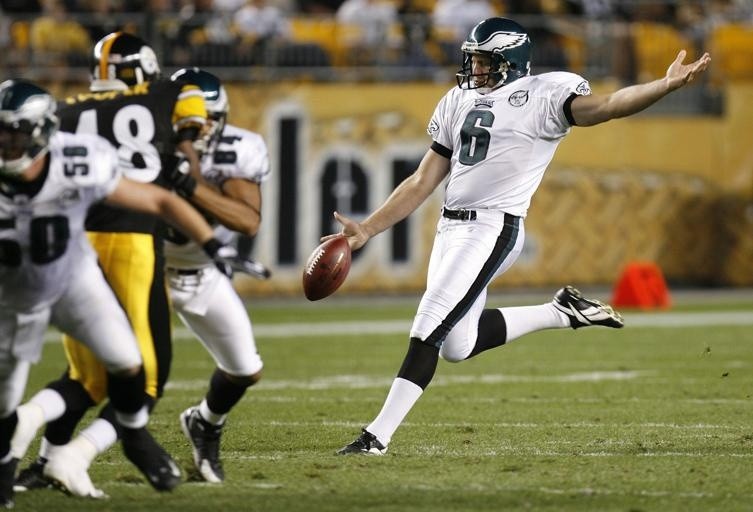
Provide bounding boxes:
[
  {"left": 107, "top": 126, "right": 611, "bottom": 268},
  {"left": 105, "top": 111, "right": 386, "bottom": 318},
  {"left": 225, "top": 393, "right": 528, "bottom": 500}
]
[
  {"left": 320, "top": 17, "right": 712, "bottom": 455},
  {"left": 16, "top": 32, "right": 207, "bottom": 500}
]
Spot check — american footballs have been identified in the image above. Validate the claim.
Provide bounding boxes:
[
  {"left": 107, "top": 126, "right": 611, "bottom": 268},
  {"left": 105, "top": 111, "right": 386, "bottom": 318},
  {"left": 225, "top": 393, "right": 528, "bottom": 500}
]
[{"left": 303, "top": 235, "right": 352, "bottom": 301}]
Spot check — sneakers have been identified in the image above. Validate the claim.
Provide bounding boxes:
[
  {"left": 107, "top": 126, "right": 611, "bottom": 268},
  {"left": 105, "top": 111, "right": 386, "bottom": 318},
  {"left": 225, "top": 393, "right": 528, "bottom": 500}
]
[
  {"left": 335, "top": 429, "right": 388, "bottom": 456},
  {"left": 44, "top": 446, "right": 108, "bottom": 500},
  {"left": 553, "top": 286, "right": 624, "bottom": 330},
  {"left": 1, "top": 409, "right": 21, "bottom": 508},
  {"left": 180, "top": 405, "right": 224, "bottom": 482},
  {"left": 120, "top": 426, "right": 180, "bottom": 492},
  {"left": 13, "top": 458, "right": 49, "bottom": 493}
]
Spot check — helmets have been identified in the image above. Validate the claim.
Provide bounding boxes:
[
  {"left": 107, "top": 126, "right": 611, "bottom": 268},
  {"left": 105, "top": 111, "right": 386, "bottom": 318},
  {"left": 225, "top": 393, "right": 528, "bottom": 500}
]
[
  {"left": 456, "top": 16, "right": 532, "bottom": 95},
  {"left": 170, "top": 68, "right": 229, "bottom": 157},
  {"left": 89, "top": 32, "right": 161, "bottom": 92},
  {"left": 1, "top": 78, "right": 60, "bottom": 173}
]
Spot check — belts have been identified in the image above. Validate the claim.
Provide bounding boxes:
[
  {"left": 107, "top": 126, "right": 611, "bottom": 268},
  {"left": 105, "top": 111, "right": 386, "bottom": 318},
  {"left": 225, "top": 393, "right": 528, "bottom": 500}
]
[
  {"left": 167, "top": 267, "right": 200, "bottom": 276},
  {"left": 442, "top": 206, "right": 476, "bottom": 221}
]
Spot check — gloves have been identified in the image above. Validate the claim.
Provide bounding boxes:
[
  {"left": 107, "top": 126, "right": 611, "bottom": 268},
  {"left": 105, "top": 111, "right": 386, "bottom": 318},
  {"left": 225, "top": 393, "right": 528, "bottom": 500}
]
[
  {"left": 203, "top": 238, "right": 270, "bottom": 278},
  {"left": 170, "top": 158, "right": 196, "bottom": 202}
]
